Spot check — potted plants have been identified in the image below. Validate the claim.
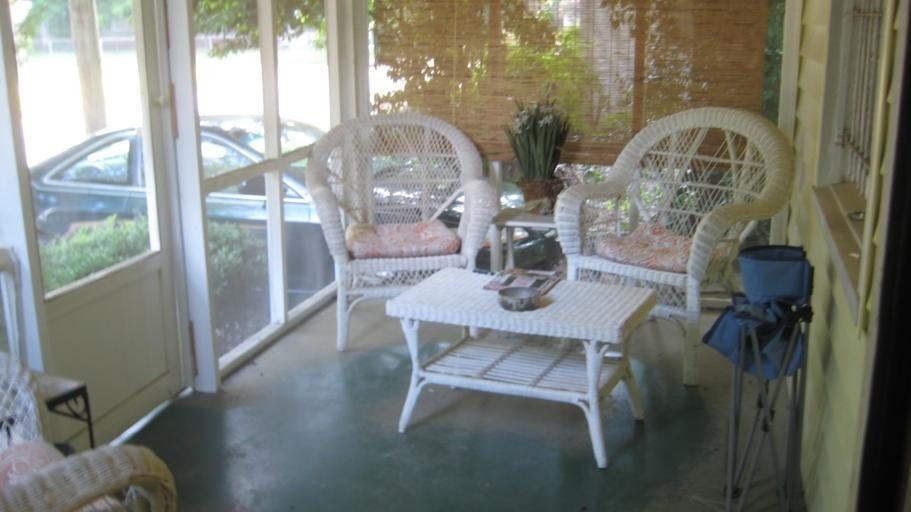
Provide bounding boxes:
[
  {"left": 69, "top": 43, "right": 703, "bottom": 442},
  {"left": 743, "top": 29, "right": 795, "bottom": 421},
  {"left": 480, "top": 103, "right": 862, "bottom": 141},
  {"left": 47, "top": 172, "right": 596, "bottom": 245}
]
[{"left": 502, "top": 88, "right": 567, "bottom": 215}]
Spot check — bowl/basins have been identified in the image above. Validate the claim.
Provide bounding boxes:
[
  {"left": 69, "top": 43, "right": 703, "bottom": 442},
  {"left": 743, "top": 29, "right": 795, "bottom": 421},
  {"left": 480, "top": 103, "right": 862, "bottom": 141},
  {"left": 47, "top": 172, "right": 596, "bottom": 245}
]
[{"left": 497, "top": 286, "right": 541, "bottom": 312}]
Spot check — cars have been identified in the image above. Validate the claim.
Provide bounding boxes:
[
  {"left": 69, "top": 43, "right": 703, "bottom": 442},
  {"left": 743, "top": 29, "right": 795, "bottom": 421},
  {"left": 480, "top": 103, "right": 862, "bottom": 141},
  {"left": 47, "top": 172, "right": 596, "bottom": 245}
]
[{"left": 30, "top": 116, "right": 564, "bottom": 308}]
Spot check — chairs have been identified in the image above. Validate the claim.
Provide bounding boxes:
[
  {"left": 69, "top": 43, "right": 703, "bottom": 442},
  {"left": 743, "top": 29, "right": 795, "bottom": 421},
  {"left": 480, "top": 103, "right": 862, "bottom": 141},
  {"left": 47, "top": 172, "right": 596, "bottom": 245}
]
[
  {"left": 553, "top": 107, "right": 795, "bottom": 384},
  {"left": 2, "top": 357, "right": 177, "bottom": 510},
  {"left": 306, "top": 113, "right": 498, "bottom": 351},
  {"left": 1, "top": 248, "right": 95, "bottom": 452}
]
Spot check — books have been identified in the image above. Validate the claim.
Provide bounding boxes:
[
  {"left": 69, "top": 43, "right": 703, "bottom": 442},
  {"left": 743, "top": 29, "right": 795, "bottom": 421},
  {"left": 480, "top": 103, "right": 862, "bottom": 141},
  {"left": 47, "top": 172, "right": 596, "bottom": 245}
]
[{"left": 482, "top": 266, "right": 568, "bottom": 297}]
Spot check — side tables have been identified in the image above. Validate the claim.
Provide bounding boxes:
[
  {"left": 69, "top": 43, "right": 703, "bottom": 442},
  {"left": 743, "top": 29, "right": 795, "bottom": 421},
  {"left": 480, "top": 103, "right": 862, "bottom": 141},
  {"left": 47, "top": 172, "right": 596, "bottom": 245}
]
[{"left": 490, "top": 203, "right": 599, "bottom": 270}]
